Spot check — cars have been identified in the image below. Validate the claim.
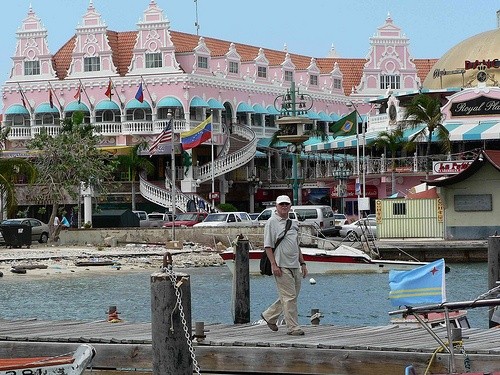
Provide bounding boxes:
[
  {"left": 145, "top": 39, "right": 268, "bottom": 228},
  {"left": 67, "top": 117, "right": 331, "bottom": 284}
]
[
  {"left": 0, "top": 218, "right": 50, "bottom": 245},
  {"left": 193, "top": 212, "right": 252, "bottom": 227},
  {"left": 339, "top": 218, "right": 377, "bottom": 242},
  {"left": 147, "top": 213, "right": 177, "bottom": 227},
  {"left": 133, "top": 211, "right": 149, "bottom": 227},
  {"left": 334, "top": 214, "right": 348, "bottom": 229},
  {"left": 162, "top": 212, "right": 208, "bottom": 228}
]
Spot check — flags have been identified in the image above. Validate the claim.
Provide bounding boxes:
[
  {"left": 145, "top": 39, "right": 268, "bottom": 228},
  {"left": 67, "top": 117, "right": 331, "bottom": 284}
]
[
  {"left": 135, "top": 83, "right": 143, "bottom": 103},
  {"left": 181, "top": 115, "right": 211, "bottom": 151},
  {"left": 331, "top": 110, "right": 357, "bottom": 139},
  {"left": 389, "top": 259, "right": 446, "bottom": 306},
  {"left": 105, "top": 81, "right": 113, "bottom": 97},
  {"left": 77, "top": 87, "right": 81, "bottom": 104},
  {"left": 149, "top": 119, "right": 172, "bottom": 158},
  {"left": 49, "top": 91, "right": 54, "bottom": 108}
]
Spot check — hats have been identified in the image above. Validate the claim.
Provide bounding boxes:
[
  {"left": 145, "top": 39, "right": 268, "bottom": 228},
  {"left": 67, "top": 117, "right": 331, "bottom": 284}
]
[{"left": 276, "top": 195, "right": 292, "bottom": 206}]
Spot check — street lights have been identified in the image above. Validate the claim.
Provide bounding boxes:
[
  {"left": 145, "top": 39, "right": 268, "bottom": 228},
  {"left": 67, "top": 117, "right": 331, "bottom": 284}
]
[
  {"left": 167, "top": 108, "right": 176, "bottom": 213},
  {"left": 346, "top": 100, "right": 377, "bottom": 199}
]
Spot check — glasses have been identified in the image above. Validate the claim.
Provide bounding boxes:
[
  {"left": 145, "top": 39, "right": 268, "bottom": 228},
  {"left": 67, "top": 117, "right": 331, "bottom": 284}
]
[{"left": 279, "top": 202, "right": 290, "bottom": 206}]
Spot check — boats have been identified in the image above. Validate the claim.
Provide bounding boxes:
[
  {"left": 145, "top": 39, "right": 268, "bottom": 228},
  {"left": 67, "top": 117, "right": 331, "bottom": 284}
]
[
  {"left": 203, "top": 231, "right": 450, "bottom": 274},
  {"left": 0, "top": 343, "right": 97, "bottom": 375}
]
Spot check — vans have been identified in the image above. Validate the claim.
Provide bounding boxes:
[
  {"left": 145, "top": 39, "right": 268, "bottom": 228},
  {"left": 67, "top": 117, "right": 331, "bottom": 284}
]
[{"left": 252, "top": 205, "right": 335, "bottom": 234}]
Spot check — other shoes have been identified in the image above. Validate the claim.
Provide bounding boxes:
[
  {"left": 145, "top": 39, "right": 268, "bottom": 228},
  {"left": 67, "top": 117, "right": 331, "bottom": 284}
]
[
  {"left": 260, "top": 314, "right": 278, "bottom": 331},
  {"left": 287, "top": 330, "right": 304, "bottom": 335}
]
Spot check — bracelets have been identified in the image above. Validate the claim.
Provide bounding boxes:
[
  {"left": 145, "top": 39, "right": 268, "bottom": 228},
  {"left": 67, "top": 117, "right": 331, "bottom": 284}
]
[{"left": 300, "top": 263, "right": 306, "bottom": 266}]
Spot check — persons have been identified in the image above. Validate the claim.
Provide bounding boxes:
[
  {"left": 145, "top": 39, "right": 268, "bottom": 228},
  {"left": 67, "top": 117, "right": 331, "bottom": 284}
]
[
  {"left": 260, "top": 195, "right": 309, "bottom": 336},
  {"left": 54, "top": 214, "right": 70, "bottom": 230}
]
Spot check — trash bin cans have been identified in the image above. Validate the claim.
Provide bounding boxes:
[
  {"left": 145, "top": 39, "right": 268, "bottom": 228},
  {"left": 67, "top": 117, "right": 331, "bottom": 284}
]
[{"left": 0, "top": 219, "right": 34, "bottom": 249}]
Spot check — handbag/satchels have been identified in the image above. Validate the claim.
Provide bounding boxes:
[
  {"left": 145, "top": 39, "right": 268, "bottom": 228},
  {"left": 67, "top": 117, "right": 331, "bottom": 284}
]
[{"left": 259, "top": 252, "right": 273, "bottom": 276}]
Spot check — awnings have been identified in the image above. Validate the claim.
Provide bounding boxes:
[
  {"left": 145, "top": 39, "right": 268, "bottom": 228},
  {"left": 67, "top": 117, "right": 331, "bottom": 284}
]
[
  {"left": 126, "top": 100, "right": 150, "bottom": 108},
  {"left": 96, "top": 100, "right": 118, "bottom": 110},
  {"left": 297, "top": 111, "right": 347, "bottom": 122},
  {"left": 36, "top": 103, "right": 59, "bottom": 112},
  {"left": 192, "top": 97, "right": 209, "bottom": 107},
  {"left": 237, "top": 103, "right": 283, "bottom": 115},
  {"left": 207, "top": 98, "right": 224, "bottom": 108},
  {"left": 5, "top": 105, "right": 29, "bottom": 114},
  {"left": 358, "top": 115, "right": 367, "bottom": 123},
  {"left": 304, "top": 118, "right": 500, "bottom": 152},
  {"left": 158, "top": 98, "right": 182, "bottom": 107},
  {"left": 64, "top": 102, "right": 88, "bottom": 111}
]
